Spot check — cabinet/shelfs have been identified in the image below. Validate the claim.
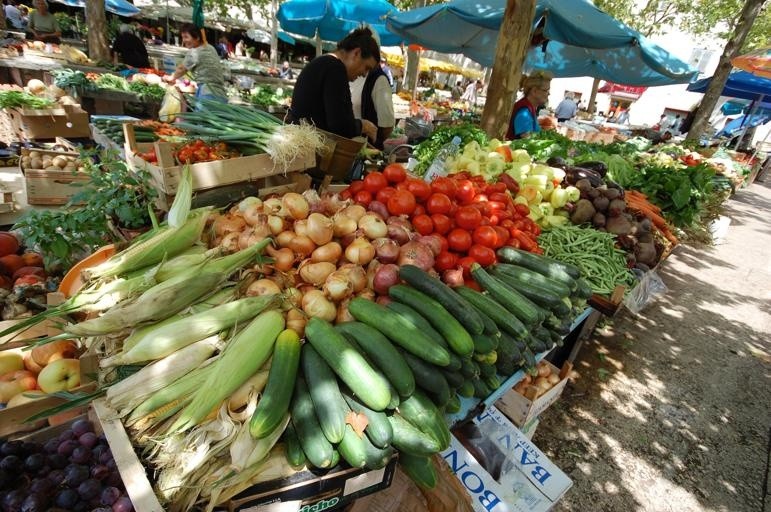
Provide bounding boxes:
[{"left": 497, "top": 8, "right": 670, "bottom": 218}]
[{"left": 121, "top": 119, "right": 318, "bottom": 222}]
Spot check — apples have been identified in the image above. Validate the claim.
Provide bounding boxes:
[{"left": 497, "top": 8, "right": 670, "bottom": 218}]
[{"left": 1, "top": 338, "right": 81, "bottom": 409}]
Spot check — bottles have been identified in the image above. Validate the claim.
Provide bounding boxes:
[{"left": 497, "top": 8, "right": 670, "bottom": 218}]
[{"left": 424, "top": 136, "right": 463, "bottom": 184}]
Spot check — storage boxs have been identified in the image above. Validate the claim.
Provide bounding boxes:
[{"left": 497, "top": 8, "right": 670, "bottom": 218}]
[
  {"left": 441, "top": 406, "right": 574, "bottom": 511},
  {"left": 122, "top": 378, "right": 398, "bottom": 512},
  {"left": 20, "top": 148, "right": 99, "bottom": 203},
  {"left": 9, "top": 103, "right": 92, "bottom": 141},
  {"left": 496, "top": 362, "right": 568, "bottom": 424}
]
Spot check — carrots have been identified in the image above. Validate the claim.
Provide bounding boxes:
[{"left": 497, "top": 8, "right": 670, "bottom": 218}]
[{"left": 625, "top": 188, "right": 677, "bottom": 247}]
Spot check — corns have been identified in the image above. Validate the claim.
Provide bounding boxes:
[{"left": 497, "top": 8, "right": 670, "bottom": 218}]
[{"left": 2, "top": 156, "right": 286, "bottom": 511}]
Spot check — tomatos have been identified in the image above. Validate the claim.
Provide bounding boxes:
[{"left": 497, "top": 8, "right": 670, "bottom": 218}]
[
  {"left": 339, "top": 162, "right": 540, "bottom": 291},
  {"left": 134, "top": 138, "right": 240, "bottom": 166}
]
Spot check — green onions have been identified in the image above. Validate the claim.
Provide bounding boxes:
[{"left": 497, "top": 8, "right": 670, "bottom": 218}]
[{"left": 162, "top": 91, "right": 330, "bottom": 179}]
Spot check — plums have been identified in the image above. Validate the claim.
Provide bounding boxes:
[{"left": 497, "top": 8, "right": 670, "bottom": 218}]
[{"left": 1, "top": 419, "right": 134, "bottom": 510}]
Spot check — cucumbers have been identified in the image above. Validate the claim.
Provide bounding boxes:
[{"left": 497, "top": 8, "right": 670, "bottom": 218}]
[
  {"left": 248, "top": 244, "right": 594, "bottom": 489},
  {"left": 92, "top": 117, "right": 158, "bottom": 148}
]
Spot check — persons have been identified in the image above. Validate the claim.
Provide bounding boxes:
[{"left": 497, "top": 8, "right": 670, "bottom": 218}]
[
  {"left": 594, "top": 101, "right": 598, "bottom": 112},
  {"left": 657, "top": 115, "right": 666, "bottom": 130},
  {"left": 518, "top": 73, "right": 528, "bottom": 102},
  {"left": 112, "top": 24, "right": 150, "bottom": 68},
  {"left": 668, "top": 113, "right": 681, "bottom": 130},
  {"left": 214, "top": 34, "right": 271, "bottom": 63},
  {"left": 161, "top": 23, "right": 228, "bottom": 112},
  {"left": 1, "top": 0, "right": 61, "bottom": 45},
  {"left": 507, "top": 69, "right": 552, "bottom": 140},
  {"left": 348, "top": 65, "right": 396, "bottom": 142},
  {"left": 289, "top": 27, "right": 380, "bottom": 146},
  {"left": 554, "top": 91, "right": 577, "bottom": 122},
  {"left": 613, "top": 107, "right": 631, "bottom": 125},
  {"left": 450, "top": 78, "right": 483, "bottom": 108}
]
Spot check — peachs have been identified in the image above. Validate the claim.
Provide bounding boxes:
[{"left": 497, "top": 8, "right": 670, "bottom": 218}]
[{"left": 0, "top": 231, "right": 54, "bottom": 291}]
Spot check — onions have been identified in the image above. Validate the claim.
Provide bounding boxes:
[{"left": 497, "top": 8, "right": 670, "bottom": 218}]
[{"left": 208, "top": 187, "right": 465, "bottom": 338}]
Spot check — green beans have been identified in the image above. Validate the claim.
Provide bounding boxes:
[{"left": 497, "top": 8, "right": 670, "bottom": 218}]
[{"left": 536, "top": 219, "right": 636, "bottom": 301}]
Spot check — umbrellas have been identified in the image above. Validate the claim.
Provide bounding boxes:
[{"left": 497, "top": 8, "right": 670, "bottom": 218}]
[
  {"left": 383, "top": 0, "right": 699, "bottom": 88},
  {"left": 686, "top": 47, "right": 771, "bottom": 151},
  {"left": 274, "top": 0, "right": 416, "bottom": 57},
  {"left": 60, "top": 0, "right": 141, "bottom": 18}
]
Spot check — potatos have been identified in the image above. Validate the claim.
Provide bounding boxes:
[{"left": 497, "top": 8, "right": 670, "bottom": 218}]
[{"left": 21, "top": 151, "right": 86, "bottom": 171}]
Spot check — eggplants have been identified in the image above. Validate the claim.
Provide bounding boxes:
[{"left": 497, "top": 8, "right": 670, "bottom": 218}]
[{"left": 545, "top": 156, "right": 625, "bottom": 198}]
